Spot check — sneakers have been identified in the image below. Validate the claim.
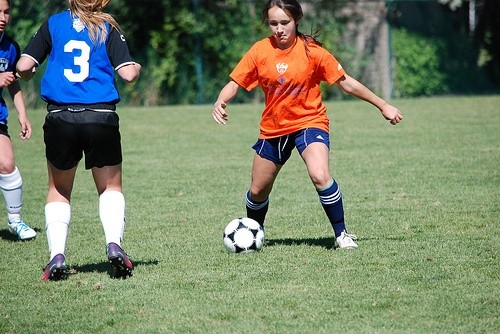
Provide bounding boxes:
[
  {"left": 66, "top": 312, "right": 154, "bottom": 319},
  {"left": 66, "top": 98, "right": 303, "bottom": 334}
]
[
  {"left": 8, "top": 219, "right": 37, "bottom": 240},
  {"left": 105, "top": 242, "right": 133, "bottom": 278},
  {"left": 41, "top": 254, "right": 68, "bottom": 281},
  {"left": 336, "top": 229, "right": 358, "bottom": 250}
]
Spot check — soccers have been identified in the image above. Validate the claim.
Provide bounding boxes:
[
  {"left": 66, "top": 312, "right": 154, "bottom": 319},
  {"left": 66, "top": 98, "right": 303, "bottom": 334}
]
[{"left": 223, "top": 216, "right": 265, "bottom": 253}]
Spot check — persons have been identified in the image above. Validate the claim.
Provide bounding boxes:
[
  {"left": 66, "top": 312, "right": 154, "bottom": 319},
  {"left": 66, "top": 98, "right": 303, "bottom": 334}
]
[
  {"left": 16, "top": 0, "right": 144, "bottom": 281},
  {"left": 212, "top": 0, "right": 403, "bottom": 248},
  {"left": 0, "top": 0, "right": 36, "bottom": 240}
]
